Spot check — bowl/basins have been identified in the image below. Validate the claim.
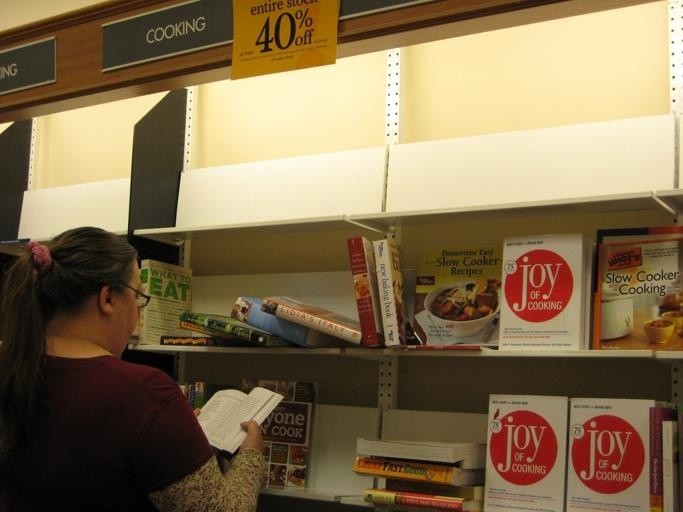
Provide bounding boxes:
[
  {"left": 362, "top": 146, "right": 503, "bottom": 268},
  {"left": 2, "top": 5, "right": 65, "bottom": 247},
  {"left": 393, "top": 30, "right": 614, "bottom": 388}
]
[
  {"left": 644, "top": 312, "right": 683, "bottom": 344},
  {"left": 424, "top": 283, "right": 501, "bottom": 338}
]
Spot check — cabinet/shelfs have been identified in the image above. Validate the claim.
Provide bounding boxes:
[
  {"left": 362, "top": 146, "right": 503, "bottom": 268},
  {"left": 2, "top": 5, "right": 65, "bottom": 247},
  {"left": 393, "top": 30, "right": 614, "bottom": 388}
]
[{"left": 133, "top": 190, "right": 682, "bottom": 511}]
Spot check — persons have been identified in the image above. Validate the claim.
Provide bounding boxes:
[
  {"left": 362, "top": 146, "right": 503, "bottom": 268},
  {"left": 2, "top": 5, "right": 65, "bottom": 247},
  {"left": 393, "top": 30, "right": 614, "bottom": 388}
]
[{"left": 0, "top": 226, "right": 266, "bottom": 512}]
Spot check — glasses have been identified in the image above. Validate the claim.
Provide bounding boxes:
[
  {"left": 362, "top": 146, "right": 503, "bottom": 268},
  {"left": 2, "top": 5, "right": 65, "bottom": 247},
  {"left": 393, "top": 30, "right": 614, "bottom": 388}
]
[{"left": 116, "top": 280, "right": 151, "bottom": 308}]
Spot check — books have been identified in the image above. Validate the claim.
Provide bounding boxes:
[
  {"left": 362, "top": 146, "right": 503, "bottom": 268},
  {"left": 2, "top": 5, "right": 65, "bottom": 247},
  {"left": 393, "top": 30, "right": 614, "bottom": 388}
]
[
  {"left": 255, "top": 379, "right": 318, "bottom": 489},
  {"left": 483, "top": 394, "right": 679, "bottom": 512},
  {"left": 197, "top": 387, "right": 284, "bottom": 454},
  {"left": 403, "top": 233, "right": 594, "bottom": 351},
  {"left": 592, "top": 225, "right": 683, "bottom": 350},
  {"left": 131, "top": 259, "right": 192, "bottom": 345},
  {"left": 180, "top": 382, "right": 240, "bottom": 410},
  {"left": 160, "top": 296, "right": 362, "bottom": 345},
  {"left": 352, "top": 436, "right": 487, "bottom": 511},
  {"left": 347, "top": 236, "right": 406, "bottom": 348}
]
